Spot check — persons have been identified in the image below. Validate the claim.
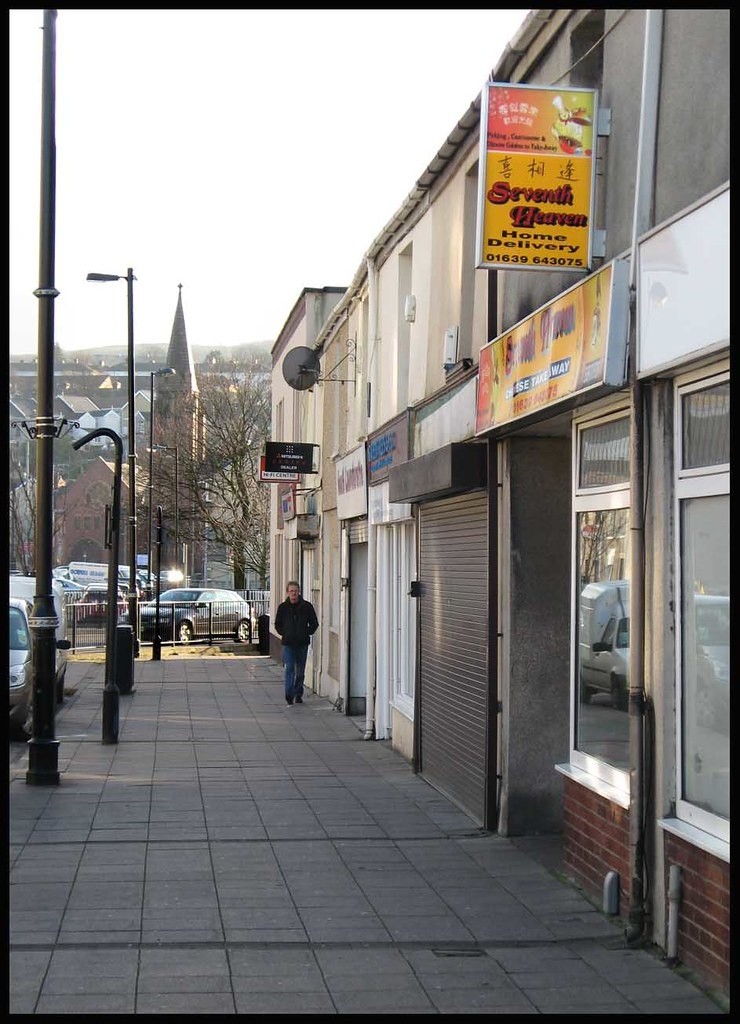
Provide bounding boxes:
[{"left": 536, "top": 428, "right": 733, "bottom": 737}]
[{"left": 275, "top": 581, "right": 319, "bottom": 705}]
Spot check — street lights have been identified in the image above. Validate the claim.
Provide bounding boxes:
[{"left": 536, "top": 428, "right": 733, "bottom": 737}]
[
  {"left": 84, "top": 267, "right": 142, "bottom": 660},
  {"left": 147, "top": 367, "right": 177, "bottom": 604}
]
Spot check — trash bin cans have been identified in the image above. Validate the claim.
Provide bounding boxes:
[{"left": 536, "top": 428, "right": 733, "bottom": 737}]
[
  {"left": 116, "top": 625, "right": 136, "bottom": 694},
  {"left": 257, "top": 614, "right": 271, "bottom": 655}
]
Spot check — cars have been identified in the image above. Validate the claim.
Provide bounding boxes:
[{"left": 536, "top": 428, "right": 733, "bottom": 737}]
[
  {"left": 9, "top": 598, "right": 34, "bottom": 741},
  {"left": 123, "top": 586, "right": 256, "bottom": 647},
  {"left": 50, "top": 561, "right": 184, "bottom": 620}
]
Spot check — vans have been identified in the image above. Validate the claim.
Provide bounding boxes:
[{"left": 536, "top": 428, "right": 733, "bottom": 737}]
[{"left": 10, "top": 576, "right": 72, "bottom": 704}]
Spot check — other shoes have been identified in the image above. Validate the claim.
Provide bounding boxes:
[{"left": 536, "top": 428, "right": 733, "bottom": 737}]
[
  {"left": 296, "top": 696, "right": 302, "bottom": 703},
  {"left": 286, "top": 695, "right": 292, "bottom": 704}
]
